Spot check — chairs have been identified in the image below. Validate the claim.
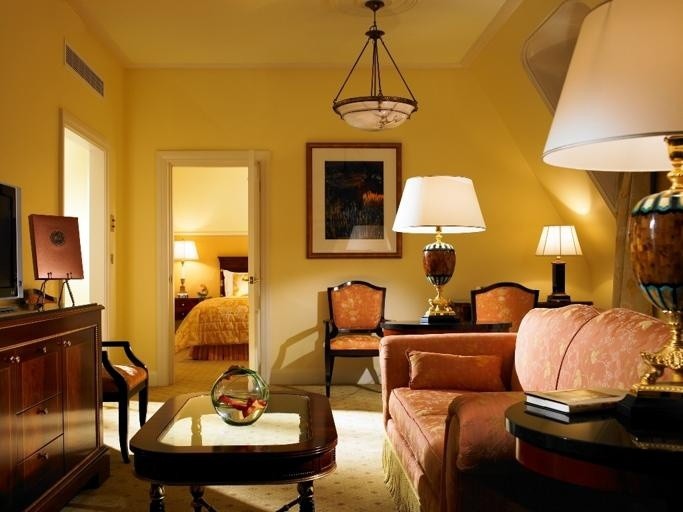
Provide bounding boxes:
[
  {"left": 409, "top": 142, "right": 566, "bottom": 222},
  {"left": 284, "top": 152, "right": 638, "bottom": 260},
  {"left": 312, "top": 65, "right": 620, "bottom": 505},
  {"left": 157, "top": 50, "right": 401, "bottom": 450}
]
[
  {"left": 102, "top": 341, "right": 149, "bottom": 464},
  {"left": 323, "top": 280, "right": 540, "bottom": 398}
]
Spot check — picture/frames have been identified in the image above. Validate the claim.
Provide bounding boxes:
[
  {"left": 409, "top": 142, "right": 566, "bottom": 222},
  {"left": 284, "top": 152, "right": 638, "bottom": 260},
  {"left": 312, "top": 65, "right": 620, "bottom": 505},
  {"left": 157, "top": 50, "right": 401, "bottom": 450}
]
[{"left": 305, "top": 142, "right": 402, "bottom": 259}]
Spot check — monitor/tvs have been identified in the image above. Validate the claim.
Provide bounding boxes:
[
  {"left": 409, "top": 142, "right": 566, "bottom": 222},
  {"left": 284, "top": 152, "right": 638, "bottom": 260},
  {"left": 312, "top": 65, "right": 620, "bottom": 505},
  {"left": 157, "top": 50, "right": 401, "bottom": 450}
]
[{"left": 0, "top": 182, "right": 23, "bottom": 313}]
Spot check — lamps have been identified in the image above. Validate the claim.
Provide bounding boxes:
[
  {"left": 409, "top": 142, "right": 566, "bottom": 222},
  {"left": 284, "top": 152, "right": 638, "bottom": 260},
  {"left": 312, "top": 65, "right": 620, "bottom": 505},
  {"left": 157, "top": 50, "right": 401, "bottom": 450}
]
[
  {"left": 535, "top": 225, "right": 593, "bottom": 308},
  {"left": 332, "top": 0, "right": 418, "bottom": 131},
  {"left": 392, "top": 175, "right": 487, "bottom": 325},
  {"left": 173, "top": 240, "right": 198, "bottom": 297},
  {"left": 541, "top": 0, "right": 683, "bottom": 432}
]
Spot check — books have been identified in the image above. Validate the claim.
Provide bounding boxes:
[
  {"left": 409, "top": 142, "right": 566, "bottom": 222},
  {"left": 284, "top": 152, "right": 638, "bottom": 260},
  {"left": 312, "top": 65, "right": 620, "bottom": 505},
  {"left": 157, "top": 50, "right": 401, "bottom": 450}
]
[
  {"left": 522, "top": 404, "right": 617, "bottom": 422},
  {"left": 524, "top": 386, "right": 622, "bottom": 413}
]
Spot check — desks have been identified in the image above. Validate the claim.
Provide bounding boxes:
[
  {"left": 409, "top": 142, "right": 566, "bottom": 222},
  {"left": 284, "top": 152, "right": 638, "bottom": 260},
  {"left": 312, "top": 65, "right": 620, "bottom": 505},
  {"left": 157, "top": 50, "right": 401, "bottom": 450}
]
[{"left": 504, "top": 389, "right": 683, "bottom": 512}]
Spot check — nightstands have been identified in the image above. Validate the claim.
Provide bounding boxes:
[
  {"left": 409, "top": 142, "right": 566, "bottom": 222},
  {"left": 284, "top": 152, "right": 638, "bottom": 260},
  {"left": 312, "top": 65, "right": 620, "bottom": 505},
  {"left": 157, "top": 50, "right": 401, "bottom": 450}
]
[{"left": 175, "top": 298, "right": 210, "bottom": 332}]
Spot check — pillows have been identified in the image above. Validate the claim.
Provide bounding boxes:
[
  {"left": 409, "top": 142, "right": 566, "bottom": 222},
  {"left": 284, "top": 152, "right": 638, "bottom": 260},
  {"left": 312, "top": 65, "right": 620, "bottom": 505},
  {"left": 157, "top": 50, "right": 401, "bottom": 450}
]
[
  {"left": 405, "top": 347, "right": 504, "bottom": 392},
  {"left": 223, "top": 270, "right": 248, "bottom": 297}
]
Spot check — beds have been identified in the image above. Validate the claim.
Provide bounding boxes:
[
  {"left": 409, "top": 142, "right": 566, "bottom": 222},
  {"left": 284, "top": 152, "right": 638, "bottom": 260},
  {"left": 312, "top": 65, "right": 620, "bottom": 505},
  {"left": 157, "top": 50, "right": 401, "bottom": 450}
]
[{"left": 174, "top": 257, "right": 249, "bottom": 361}]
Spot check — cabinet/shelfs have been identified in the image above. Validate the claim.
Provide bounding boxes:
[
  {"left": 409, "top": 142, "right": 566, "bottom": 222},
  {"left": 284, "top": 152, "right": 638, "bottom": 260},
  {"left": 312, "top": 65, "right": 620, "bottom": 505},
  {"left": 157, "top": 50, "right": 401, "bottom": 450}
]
[{"left": 0, "top": 303, "right": 112, "bottom": 512}]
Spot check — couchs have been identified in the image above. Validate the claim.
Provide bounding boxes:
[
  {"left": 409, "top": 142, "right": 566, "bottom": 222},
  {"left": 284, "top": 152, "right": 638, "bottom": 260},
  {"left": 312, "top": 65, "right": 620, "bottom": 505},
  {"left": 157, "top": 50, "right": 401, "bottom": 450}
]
[{"left": 378, "top": 304, "right": 683, "bottom": 512}]
